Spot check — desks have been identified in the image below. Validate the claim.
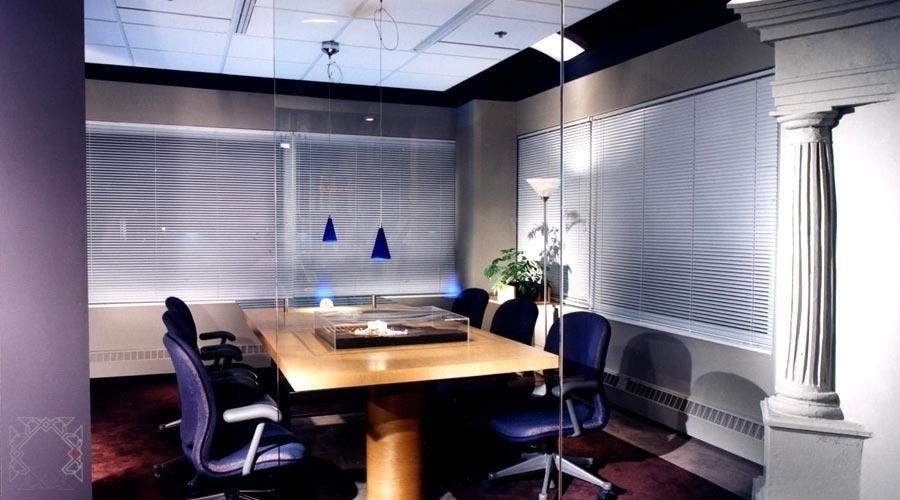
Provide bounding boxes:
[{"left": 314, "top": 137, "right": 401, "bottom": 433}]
[{"left": 238, "top": 304, "right": 558, "bottom": 500}]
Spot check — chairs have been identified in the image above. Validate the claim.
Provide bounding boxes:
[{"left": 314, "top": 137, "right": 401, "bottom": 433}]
[
  {"left": 435, "top": 298, "right": 538, "bottom": 428},
  {"left": 158, "top": 311, "right": 281, "bottom": 432},
  {"left": 451, "top": 288, "right": 488, "bottom": 328},
  {"left": 154, "top": 333, "right": 310, "bottom": 499},
  {"left": 166, "top": 297, "right": 259, "bottom": 379},
  {"left": 487, "top": 311, "right": 615, "bottom": 500}
]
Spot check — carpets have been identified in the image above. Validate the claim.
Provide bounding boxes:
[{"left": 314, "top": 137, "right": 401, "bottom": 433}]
[{"left": 90, "top": 371, "right": 748, "bottom": 500}]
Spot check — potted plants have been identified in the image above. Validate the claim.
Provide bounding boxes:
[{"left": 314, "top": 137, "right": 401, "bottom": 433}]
[{"left": 483, "top": 247, "right": 552, "bottom": 302}]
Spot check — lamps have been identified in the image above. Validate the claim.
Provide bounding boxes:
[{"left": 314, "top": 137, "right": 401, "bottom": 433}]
[
  {"left": 321, "top": 40, "right": 338, "bottom": 241},
  {"left": 525, "top": 178, "right": 559, "bottom": 303},
  {"left": 372, "top": 0, "right": 390, "bottom": 259}
]
